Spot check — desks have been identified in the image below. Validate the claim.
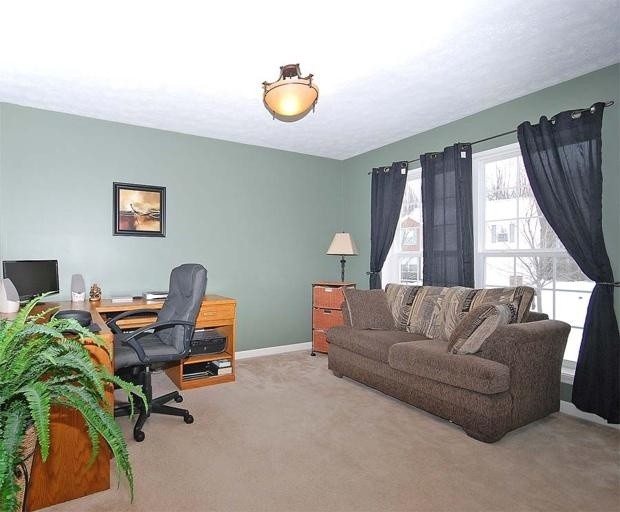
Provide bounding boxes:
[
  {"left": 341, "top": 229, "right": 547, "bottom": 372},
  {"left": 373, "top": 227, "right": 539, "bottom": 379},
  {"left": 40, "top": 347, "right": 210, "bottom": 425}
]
[{"left": 0, "top": 293, "right": 237, "bottom": 512}]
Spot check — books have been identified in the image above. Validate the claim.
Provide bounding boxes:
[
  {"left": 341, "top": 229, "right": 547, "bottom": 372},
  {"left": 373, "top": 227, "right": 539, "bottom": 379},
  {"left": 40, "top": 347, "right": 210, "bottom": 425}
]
[
  {"left": 111, "top": 295, "right": 133, "bottom": 303},
  {"left": 179, "top": 359, "right": 233, "bottom": 381}
]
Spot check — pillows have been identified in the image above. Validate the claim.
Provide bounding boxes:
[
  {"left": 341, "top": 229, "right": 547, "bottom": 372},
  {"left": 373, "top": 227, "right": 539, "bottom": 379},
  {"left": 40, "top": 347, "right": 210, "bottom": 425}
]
[
  {"left": 446, "top": 297, "right": 521, "bottom": 357},
  {"left": 342, "top": 286, "right": 396, "bottom": 330}
]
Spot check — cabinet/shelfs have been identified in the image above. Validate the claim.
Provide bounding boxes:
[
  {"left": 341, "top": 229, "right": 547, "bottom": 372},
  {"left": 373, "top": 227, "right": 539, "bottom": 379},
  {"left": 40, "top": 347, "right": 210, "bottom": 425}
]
[{"left": 313, "top": 280, "right": 355, "bottom": 354}]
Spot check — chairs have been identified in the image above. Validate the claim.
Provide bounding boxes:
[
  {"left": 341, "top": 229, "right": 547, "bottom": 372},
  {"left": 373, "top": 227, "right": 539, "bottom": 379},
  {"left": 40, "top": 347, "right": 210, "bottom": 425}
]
[{"left": 104, "top": 263, "right": 207, "bottom": 441}]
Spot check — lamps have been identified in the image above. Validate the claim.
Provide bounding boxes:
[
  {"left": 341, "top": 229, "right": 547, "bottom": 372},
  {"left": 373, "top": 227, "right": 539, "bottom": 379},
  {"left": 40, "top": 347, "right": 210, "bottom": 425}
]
[
  {"left": 261, "top": 64, "right": 318, "bottom": 118},
  {"left": 327, "top": 231, "right": 360, "bottom": 282}
]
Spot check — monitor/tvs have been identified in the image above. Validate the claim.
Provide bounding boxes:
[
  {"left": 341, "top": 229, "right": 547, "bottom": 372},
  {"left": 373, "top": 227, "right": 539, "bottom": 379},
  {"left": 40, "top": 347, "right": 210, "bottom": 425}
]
[{"left": 2, "top": 259, "right": 60, "bottom": 307}]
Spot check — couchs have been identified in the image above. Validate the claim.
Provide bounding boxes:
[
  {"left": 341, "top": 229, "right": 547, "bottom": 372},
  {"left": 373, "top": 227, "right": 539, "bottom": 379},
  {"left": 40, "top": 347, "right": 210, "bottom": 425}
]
[{"left": 325, "top": 282, "right": 571, "bottom": 444}]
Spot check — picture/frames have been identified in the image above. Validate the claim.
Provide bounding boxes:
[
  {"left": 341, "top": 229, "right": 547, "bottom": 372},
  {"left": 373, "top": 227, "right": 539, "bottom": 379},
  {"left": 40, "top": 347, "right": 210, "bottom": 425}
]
[{"left": 113, "top": 180, "right": 168, "bottom": 238}]
